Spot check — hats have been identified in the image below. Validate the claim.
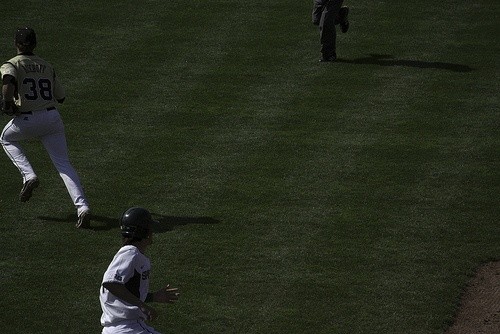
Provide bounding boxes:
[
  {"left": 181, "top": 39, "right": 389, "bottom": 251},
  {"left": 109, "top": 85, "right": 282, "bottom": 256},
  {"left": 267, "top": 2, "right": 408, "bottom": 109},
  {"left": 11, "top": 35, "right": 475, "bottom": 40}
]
[{"left": 15, "top": 27, "right": 36, "bottom": 45}]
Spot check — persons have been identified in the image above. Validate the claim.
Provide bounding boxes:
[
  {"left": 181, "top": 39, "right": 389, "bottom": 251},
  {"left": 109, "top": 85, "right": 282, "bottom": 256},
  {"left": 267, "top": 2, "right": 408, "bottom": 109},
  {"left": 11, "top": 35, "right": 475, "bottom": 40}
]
[
  {"left": 0, "top": 26, "right": 92, "bottom": 229},
  {"left": 98, "top": 207, "right": 181, "bottom": 334},
  {"left": 312, "top": 0, "right": 349, "bottom": 63}
]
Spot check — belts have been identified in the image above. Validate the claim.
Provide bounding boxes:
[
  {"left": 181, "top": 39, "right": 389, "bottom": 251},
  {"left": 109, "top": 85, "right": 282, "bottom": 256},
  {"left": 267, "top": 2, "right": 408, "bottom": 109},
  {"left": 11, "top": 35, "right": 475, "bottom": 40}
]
[{"left": 21, "top": 106, "right": 56, "bottom": 114}]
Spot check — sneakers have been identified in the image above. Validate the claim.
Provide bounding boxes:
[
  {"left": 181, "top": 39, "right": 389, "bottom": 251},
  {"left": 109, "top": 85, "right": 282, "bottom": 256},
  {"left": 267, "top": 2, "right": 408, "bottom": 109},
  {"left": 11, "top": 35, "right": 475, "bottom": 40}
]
[
  {"left": 20, "top": 178, "right": 39, "bottom": 202},
  {"left": 75, "top": 210, "right": 92, "bottom": 229},
  {"left": 340, "top": 5, "right": 349, "bottom": 33},
  {"left": 319, "top": 52, "right": 336, "bottom": 62}
]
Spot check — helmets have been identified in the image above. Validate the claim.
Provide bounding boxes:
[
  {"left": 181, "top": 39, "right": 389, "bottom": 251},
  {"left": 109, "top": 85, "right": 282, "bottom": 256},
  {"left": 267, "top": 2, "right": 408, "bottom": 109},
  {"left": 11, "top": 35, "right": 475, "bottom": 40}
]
[{"left": 120, "top": 207, "right": 160, "bottom": 236}]
